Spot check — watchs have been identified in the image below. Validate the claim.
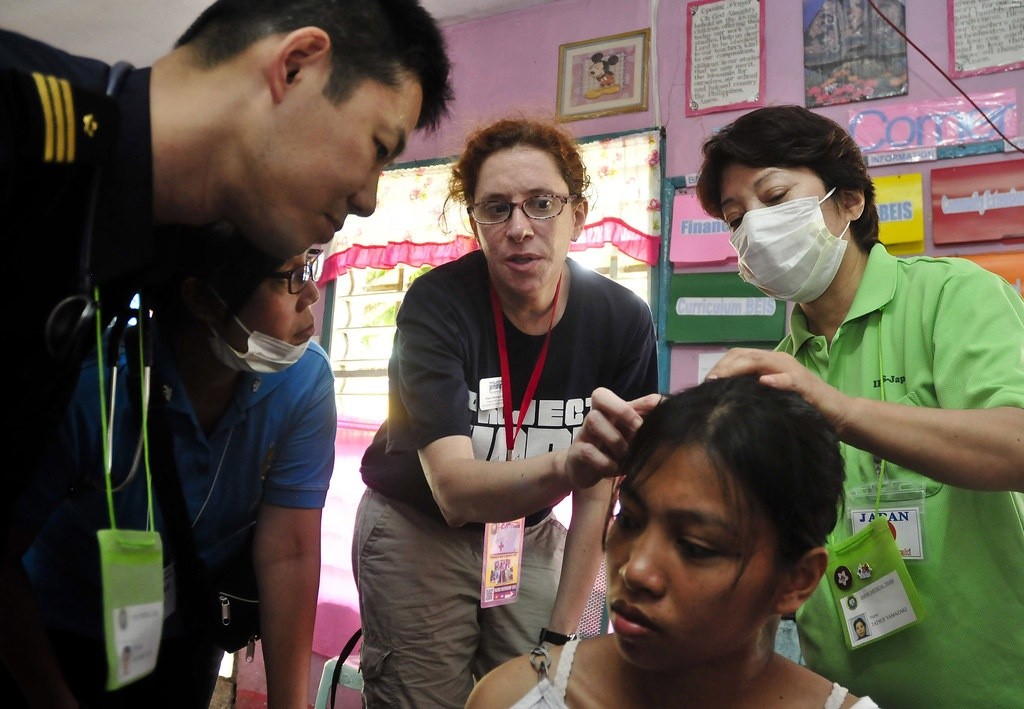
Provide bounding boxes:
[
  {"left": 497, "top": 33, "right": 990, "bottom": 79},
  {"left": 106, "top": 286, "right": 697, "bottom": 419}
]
[{"left": 539, "top": 627, "right": 578, "bottom": 646}]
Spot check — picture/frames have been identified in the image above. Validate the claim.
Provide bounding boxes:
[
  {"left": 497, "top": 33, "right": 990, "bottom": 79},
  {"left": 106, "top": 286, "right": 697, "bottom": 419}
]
[{"left": 555, "top": 27, "right": 650, "bottom": 122}]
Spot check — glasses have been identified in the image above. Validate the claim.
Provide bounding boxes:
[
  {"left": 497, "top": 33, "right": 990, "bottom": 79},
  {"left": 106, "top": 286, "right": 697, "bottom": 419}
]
[
  {"left": 467, "top": 194, "right": 580, "bottom": 226},
  {"left": 270, "top": 248, "right": 325, "bottom": 295}
]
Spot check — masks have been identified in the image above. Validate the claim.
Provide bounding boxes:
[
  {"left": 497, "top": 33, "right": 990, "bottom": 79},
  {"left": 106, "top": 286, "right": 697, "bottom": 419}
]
[
  {"left": 727, "top": 187, "right": 851, "bottom": 305},
  {"left": 195, "top": 288, "right": 311, "bottom": 375}
]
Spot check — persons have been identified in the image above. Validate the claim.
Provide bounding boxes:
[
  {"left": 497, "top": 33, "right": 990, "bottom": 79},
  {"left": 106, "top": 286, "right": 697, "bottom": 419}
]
[
  {"left": 696, "top": 104, "right": 1024, "bottom": 709},
  {"left": 1, "top": 0, "right": 456, "bottom": 709},
  {"left": 350, "top": 118, "right": 669, "bottom": 709},
  {"left": 463, "top": 370, "right": 880, "bottom": 707},
  {"left": 166, "top": 248, "right": 337, "bottom": 709}
]
